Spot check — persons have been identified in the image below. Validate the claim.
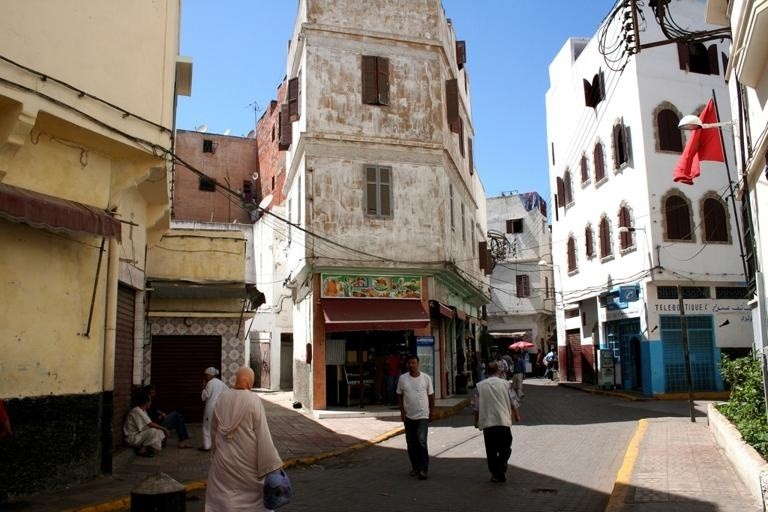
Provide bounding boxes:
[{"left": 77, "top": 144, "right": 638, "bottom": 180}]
[
  {"left": 196, "top": 366, "right": 233, "bottom": 452},
  {"left": 143, "top": 383, "right": 195, "bottom": 449},
  {"left": 396, "top": 353, "right": 435, "bottom": 480},
  {"left": 383, "top": 344, "right": 404, "bottom": 404},
  {"left": 481, "top": 348, "right": 559, "bottom": 402},
  {"left": 204, "top": 365, "right": 284, "bottom": 512},
  {"left": 470, "top": 361, "right": 521, "bottom": 484},
  {"left": 122, "top": 393, "right": 172, "bottom": 457}
]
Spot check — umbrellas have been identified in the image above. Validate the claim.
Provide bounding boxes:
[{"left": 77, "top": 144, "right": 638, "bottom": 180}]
[{"left": 508, "top": 341, "right": 536, "bottom": 349}]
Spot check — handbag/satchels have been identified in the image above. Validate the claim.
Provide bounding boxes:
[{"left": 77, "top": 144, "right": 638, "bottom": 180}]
[{"left": 263, "top": 469, "right": 293, "bottom": 509}]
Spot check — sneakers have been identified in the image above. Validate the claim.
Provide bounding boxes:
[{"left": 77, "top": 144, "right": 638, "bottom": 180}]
[{"left": 492, "top": 474, "right": 507, "bottom": 483}]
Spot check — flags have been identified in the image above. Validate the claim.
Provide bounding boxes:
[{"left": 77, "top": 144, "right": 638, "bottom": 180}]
[{"left": 671, "top": 95, "right": 725, "bottom": 187}]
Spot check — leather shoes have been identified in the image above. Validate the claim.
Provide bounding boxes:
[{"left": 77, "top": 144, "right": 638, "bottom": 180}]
[
  {"left": 419, "top": 472, "right": 428, "bottom": 480},
  {"left": 410, "top": 470, "right": 417, "bottom": 477}
]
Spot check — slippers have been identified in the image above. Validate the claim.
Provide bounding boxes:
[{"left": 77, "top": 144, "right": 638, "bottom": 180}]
[{"left": 138, "top": 439, "right": 208, "bottom": 458}]
